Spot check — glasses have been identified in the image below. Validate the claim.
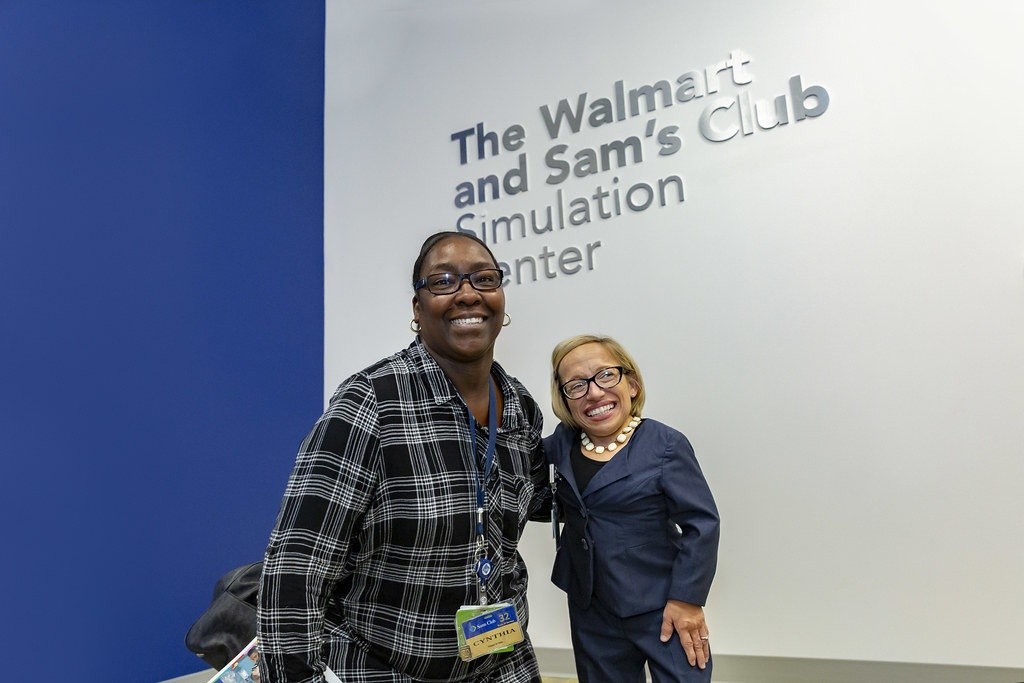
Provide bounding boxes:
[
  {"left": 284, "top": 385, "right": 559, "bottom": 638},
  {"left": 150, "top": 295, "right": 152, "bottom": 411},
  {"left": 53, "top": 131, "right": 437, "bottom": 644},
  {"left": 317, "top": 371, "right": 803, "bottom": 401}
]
[
  {"left": 416, "top": 269, "right": 504, "bottom": 295},
  {"left": 558, "top": 366, "right": 630, "bottom": 400}
]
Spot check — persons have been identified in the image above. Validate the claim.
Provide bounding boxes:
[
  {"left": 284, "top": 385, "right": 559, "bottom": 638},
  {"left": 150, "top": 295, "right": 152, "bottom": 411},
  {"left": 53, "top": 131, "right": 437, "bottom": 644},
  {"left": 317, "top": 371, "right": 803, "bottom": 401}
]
[
  {"left": 249, "top": 646, "right": 260, "bottom": 683},
  {"left": 543, "top": 335, "right": 721, "bottom": 683},
  {"left": 256, "top": 233, "right": 560, "bottom": 683}
]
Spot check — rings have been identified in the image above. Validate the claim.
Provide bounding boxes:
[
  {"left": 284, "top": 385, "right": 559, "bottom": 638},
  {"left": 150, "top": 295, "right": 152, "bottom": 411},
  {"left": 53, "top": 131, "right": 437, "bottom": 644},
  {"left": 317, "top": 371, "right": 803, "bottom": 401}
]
[{"left": 701, "top": 636, "right": 708, "bottom": 639}]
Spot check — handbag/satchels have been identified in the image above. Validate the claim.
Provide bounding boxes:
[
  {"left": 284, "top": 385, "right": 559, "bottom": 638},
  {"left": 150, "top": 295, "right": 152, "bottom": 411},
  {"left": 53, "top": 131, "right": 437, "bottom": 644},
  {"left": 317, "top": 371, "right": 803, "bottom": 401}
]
[{"left": 185, "top": 560, "right": 264, "bottom": 671}]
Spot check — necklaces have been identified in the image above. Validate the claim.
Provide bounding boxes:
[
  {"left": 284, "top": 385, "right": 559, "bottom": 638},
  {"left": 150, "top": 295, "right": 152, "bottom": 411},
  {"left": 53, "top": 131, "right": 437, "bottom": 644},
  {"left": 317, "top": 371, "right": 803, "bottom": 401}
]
[{"left": 580, "top": 417, "right": 641, "bottom": 454}]
